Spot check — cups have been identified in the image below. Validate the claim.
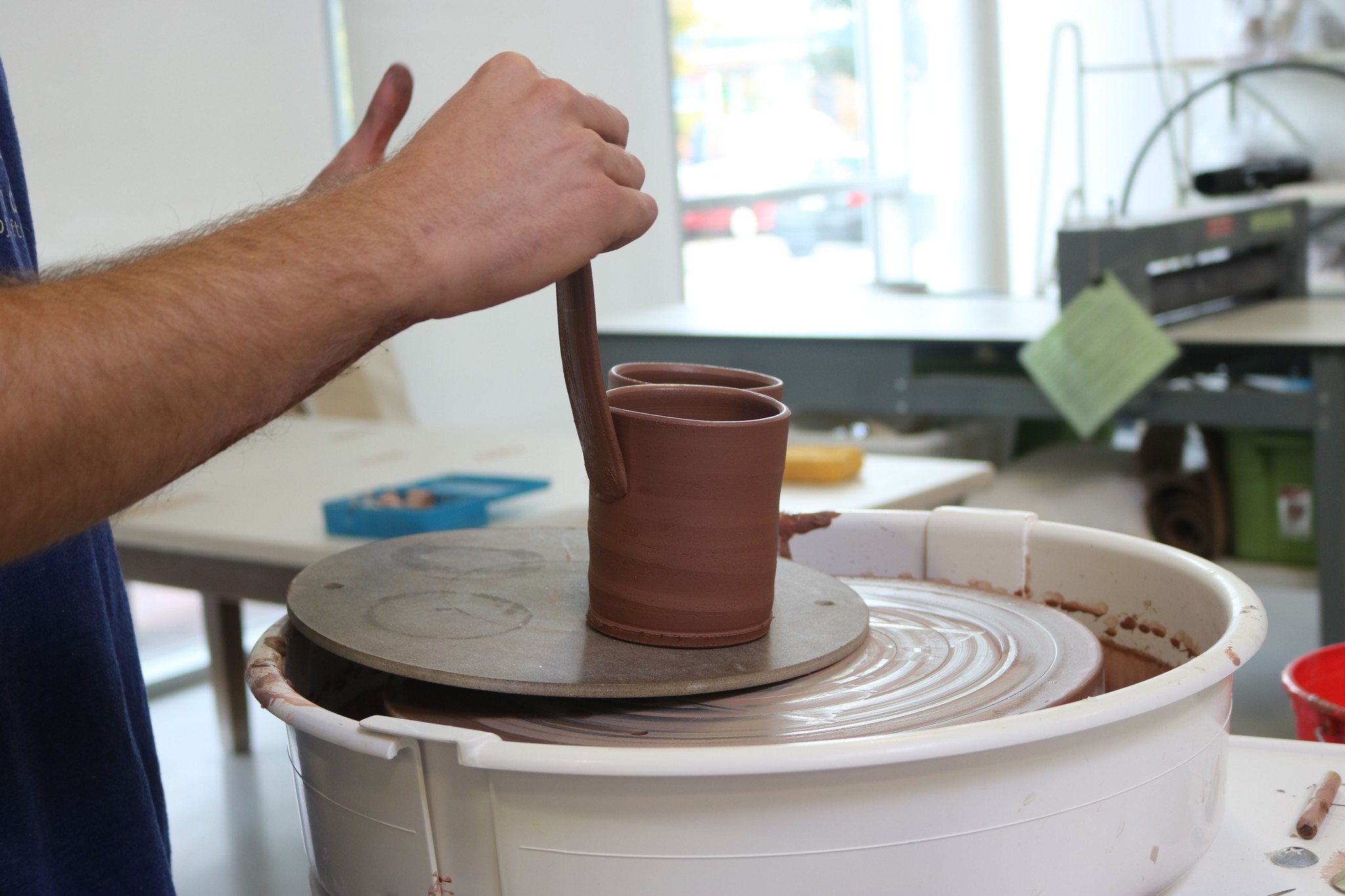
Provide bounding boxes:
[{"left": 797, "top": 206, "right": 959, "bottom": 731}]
[
  {"left": 608, "top": 361, "right": 783, "bottom": 402},
  {"left": 591, "top": 383, "right": 789, "bottom": 647}
]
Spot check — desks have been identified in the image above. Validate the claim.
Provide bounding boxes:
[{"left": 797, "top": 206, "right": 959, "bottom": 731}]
[
  {"left": 109, "top": 416, "right": 997, "bottom": 756},
  {"left": 587, "top": 294, "right": 1345, "bottom": 647}
]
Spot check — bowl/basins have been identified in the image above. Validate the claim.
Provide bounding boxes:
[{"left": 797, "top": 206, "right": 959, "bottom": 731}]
[{"left": 243, "top": 503, "right": 1269, "bottom": 896}]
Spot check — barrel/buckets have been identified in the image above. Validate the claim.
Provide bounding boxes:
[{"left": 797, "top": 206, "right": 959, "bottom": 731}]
[{"left": 1281, "top": 642, "right": 1345, "bottom": 744}]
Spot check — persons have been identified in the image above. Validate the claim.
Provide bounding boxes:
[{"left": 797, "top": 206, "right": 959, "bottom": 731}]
[{"left": 1, "top": 50, "right": 658, "bottom": 896}]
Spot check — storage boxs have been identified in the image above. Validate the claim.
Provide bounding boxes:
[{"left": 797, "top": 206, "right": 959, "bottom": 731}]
[
  {"left": 321, "top": 476, "right": 550, "bottom": 538},
  {"left": 1231, "top": 431, "right": 1320, "bottom": 563}
]
